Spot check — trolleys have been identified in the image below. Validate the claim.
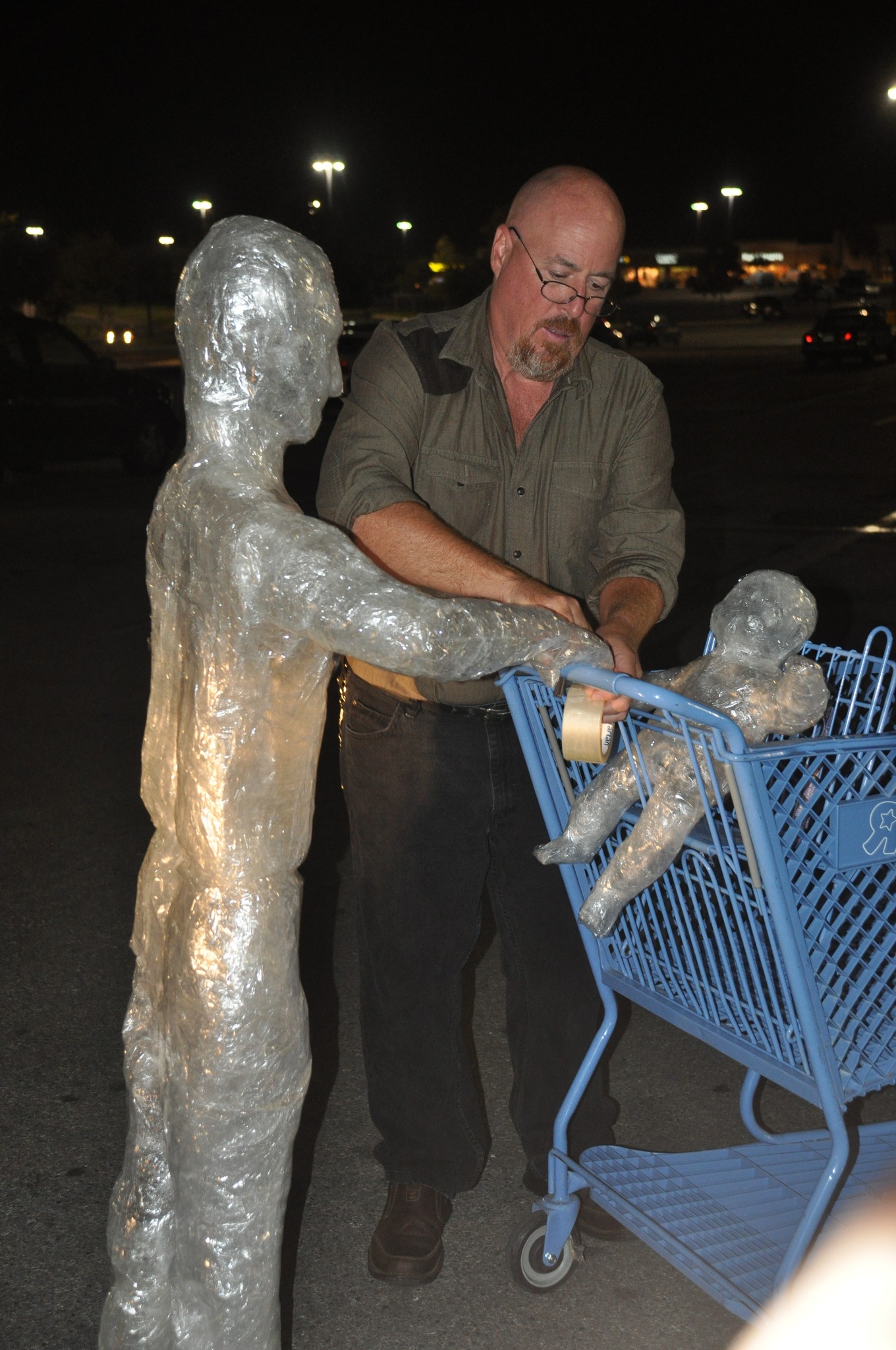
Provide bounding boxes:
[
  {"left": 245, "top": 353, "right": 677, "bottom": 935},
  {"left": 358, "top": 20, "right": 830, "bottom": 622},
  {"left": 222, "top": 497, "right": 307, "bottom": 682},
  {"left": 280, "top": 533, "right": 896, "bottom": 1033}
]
[{"left": 495, "top": 622, "right": 896, "bottom": 1320}]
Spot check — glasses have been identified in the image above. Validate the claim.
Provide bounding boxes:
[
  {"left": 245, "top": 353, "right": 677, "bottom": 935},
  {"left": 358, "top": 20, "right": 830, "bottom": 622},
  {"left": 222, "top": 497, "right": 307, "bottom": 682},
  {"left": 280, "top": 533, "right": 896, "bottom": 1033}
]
[{"left": 507, "top": 225, "right": 620, "bottom": 318}]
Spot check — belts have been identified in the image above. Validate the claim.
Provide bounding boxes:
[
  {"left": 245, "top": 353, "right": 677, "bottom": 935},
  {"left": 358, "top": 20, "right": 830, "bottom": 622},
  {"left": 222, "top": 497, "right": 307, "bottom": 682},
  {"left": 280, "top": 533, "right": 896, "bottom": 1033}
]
[{"left": 443, "top": 704, "right": 511, "bottom": 720}]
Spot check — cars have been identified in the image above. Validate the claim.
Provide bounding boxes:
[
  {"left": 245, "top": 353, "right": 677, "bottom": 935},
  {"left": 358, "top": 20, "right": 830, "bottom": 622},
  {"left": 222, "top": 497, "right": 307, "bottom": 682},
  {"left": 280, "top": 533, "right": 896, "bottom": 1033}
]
[
  {"left": 801, "top": 306, "right": 896, "bottom": 370},
  {"left": 591, "top": 303, "right": 661, "bottom": 349},
  {"left": 0, "top": 305, "right": 180, "bottom": 478},
  {"left": 837, "top": 268, "right": 880, "bottom": 298}
]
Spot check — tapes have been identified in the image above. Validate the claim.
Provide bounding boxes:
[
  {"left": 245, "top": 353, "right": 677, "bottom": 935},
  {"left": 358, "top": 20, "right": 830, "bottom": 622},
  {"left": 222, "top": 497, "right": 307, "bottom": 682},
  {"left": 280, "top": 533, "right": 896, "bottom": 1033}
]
[{"left": 561, "top": 681, "right": 616, "bottom": 763}]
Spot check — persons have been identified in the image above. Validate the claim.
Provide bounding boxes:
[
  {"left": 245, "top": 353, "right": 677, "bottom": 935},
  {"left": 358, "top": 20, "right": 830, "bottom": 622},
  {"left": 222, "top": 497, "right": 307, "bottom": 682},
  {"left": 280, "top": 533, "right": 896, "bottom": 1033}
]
[
  {"left": 316, "top": 164, "right": 683, "bottom": 1285},
  {"left": 532, "top": 572, "right": 828, "bottom": 929},
  {"left": 94, "top": 219, "right": 611, "bottom": 1350}
]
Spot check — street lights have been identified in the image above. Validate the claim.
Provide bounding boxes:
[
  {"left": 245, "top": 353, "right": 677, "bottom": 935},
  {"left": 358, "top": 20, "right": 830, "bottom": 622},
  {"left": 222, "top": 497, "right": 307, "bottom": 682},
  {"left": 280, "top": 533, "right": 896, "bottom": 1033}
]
[
  {"left": 311, "top": 158, "right": 347, "bottom": 210},
  {"left": 720, "top": 186, "right": 743, "bottom": 227},
  {"left": 193, "top": 200, "right": 212, "bottom": 238}
]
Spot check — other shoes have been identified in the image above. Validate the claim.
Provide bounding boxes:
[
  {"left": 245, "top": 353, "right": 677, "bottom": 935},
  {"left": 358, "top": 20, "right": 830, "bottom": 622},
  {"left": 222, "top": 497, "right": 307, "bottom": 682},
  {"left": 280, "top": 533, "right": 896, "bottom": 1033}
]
[
  {"left": 523, "top": 1142, "right": 642, "bottom": 1241},
  {"left": 367, "top": 1182, "right": 452, "bottom": 1285}
]
